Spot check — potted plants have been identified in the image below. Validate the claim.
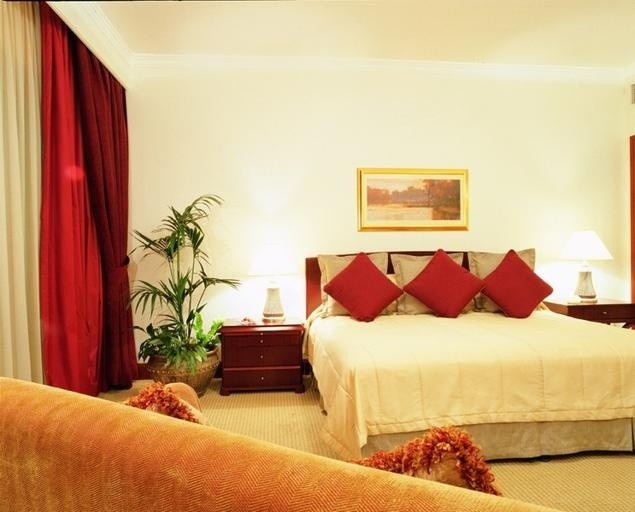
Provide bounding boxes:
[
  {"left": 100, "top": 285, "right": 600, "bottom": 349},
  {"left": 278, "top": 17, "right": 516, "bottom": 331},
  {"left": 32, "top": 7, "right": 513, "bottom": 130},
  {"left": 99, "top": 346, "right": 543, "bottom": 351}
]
[{"left": 125, "top": 192, "right": 241, "bottom": 397}]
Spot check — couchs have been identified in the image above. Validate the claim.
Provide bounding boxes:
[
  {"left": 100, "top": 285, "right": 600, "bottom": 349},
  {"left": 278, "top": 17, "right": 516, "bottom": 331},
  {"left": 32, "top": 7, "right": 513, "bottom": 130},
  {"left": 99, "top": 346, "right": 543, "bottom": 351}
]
[{"left": 0, "top": 372, "right": 560, "bottom": 511}]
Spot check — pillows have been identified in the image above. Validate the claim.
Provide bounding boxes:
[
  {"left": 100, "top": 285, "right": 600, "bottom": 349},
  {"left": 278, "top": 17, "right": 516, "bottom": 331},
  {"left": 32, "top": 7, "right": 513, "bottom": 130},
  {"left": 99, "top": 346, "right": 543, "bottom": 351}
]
[
  {"left": 122, "top": 384, "right": 212, "bottom": 425},
  {"left": 317, "top": 250, "right": 554, "bottom": 322},
  {"left": 342, "top": 423, "right": 505, "bottom": 496}
]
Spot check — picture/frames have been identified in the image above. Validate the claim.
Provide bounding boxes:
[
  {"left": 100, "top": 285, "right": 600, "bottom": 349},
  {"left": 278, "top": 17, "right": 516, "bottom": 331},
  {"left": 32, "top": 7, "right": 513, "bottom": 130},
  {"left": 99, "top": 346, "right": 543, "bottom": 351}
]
[{"left": 357, "top": 167, "right": 470, "bottom": 230}]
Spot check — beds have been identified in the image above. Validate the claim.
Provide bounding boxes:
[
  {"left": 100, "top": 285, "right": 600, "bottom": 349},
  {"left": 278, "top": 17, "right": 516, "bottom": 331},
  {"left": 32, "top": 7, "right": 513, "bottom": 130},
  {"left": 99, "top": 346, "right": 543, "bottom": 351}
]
[{"left": 303, "top": 248, "right": 635, "bottom": 462}]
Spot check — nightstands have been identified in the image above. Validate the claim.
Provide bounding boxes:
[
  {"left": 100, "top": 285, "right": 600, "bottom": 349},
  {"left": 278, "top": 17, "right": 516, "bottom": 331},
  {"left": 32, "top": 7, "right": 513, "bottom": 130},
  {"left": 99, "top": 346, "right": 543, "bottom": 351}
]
[
  {"left": 220, "top": 327, "right": 305, "bottom": 394},
  {"left": 543, "top": 297, "right": 634, "bottom": 327}
]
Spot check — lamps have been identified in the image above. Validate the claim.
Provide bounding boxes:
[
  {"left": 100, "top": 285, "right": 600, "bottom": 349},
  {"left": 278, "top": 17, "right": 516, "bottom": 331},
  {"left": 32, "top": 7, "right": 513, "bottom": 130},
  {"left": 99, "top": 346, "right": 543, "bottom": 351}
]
[
  {"left": 555, "top": 230, "right": 615, "bottom": 302},
  {"left": 248, "top": 223, "right": 302, "bottom": 325}
]
